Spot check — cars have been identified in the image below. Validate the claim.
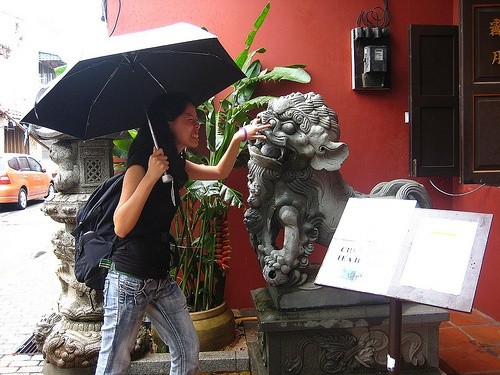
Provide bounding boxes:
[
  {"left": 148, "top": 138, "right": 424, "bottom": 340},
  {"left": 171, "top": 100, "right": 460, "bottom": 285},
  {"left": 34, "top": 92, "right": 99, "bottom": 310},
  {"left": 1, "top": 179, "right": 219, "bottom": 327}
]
[{"left": 0, "top": 152, "right": 55, "bottom": 210}]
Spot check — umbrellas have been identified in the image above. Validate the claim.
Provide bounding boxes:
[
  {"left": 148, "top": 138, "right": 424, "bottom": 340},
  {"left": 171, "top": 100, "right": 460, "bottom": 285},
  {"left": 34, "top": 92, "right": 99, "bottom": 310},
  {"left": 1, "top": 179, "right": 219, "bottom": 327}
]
[{"left": 15, "top": 21, "right": 248, "bottom": 186}]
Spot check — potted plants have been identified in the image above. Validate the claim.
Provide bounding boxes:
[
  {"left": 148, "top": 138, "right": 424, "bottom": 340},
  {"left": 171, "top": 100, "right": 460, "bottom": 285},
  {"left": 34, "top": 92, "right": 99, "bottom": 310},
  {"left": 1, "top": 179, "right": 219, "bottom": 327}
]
[{"left": 112, "top": 0, "right": 311, "bottom": 353}]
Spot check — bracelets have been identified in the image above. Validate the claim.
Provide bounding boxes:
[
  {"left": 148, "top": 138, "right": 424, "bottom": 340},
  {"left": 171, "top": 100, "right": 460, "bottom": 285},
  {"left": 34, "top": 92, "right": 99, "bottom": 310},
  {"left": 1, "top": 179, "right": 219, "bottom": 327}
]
[{"left": 236, "top": 127, "right": 249, "bottom": 142}]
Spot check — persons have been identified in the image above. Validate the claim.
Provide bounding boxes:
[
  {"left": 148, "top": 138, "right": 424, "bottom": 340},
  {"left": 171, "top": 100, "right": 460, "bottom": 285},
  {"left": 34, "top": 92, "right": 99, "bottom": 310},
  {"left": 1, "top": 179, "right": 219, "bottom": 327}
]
[{"left": 67, "top": 99, "right": 272, "bottom": 375}]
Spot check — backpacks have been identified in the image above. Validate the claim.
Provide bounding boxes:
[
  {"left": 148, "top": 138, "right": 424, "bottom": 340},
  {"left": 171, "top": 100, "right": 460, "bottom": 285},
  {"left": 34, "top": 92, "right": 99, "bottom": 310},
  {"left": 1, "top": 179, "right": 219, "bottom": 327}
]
[{"left": 71, "top": 159, "right": 151, "bottom": 288}]
